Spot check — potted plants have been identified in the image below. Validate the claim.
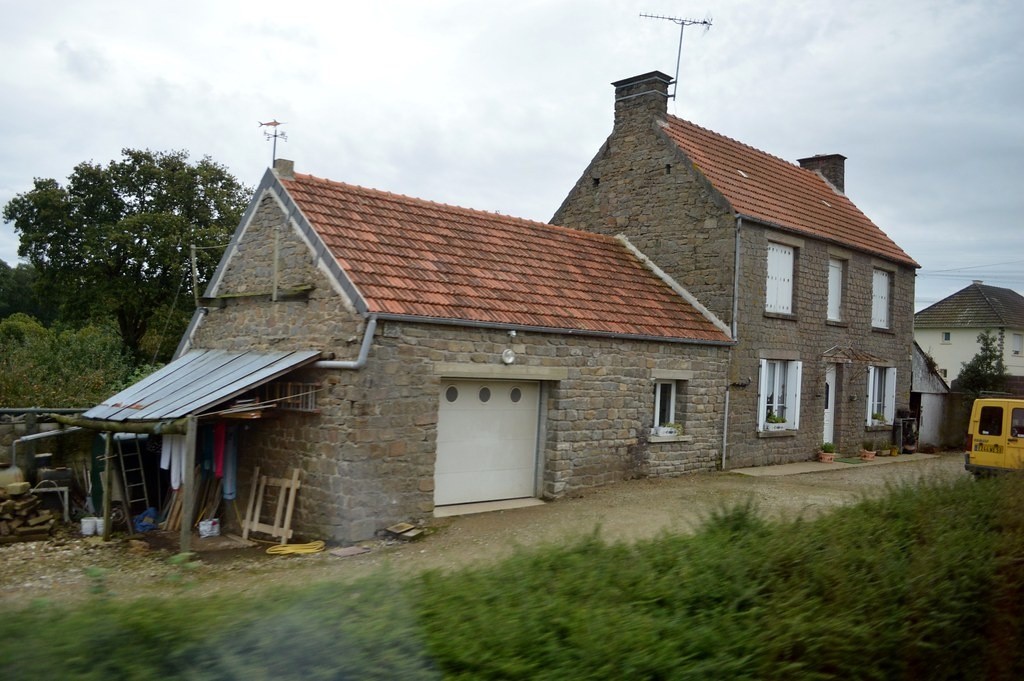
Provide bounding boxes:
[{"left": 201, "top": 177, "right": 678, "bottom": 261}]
[
  {"left": 80, "top": 517, "right": 113, "bottom": 536},
  {"left": 656, "top": 423, "right": 684, "bottom": 438},
  {"left": 897, "top": 404, "right": 917, "bottom": 454},
  {"left": 871, "top": 413, "right": 888, "bottom": 426},
  {"left": 764, "top": 414, "right": 788, "bottom": 431},
  {"left": 859, "top": 438, "right": 900, "bottom": 460},
  {"left": 818, "top": 442, "right": 836, "bottom": 462}
]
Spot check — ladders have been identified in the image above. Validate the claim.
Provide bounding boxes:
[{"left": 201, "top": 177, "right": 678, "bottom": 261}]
[
  {"left": 265, "top": 381, "right": 321, "bottom": 414},
  {"left": 116, "top": 434, "right": 150, "bottom": 516}
]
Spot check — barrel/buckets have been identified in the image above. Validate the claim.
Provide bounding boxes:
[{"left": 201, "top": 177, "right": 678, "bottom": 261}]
[
  {"left": 81, "top": 519, "right": 96, "bottom": 535},
  {"left": 96, "top": 520, "right": 103, "bottom": 536}
]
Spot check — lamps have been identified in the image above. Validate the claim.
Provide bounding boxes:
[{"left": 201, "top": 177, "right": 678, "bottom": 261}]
[{"left": 502, "top": 349, "right": 516, "bottom": 364}]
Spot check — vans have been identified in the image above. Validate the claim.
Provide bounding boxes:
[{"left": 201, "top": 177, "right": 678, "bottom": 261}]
[{"left": 964, "top": 398, "right": 1024, "bottom": 474}]
